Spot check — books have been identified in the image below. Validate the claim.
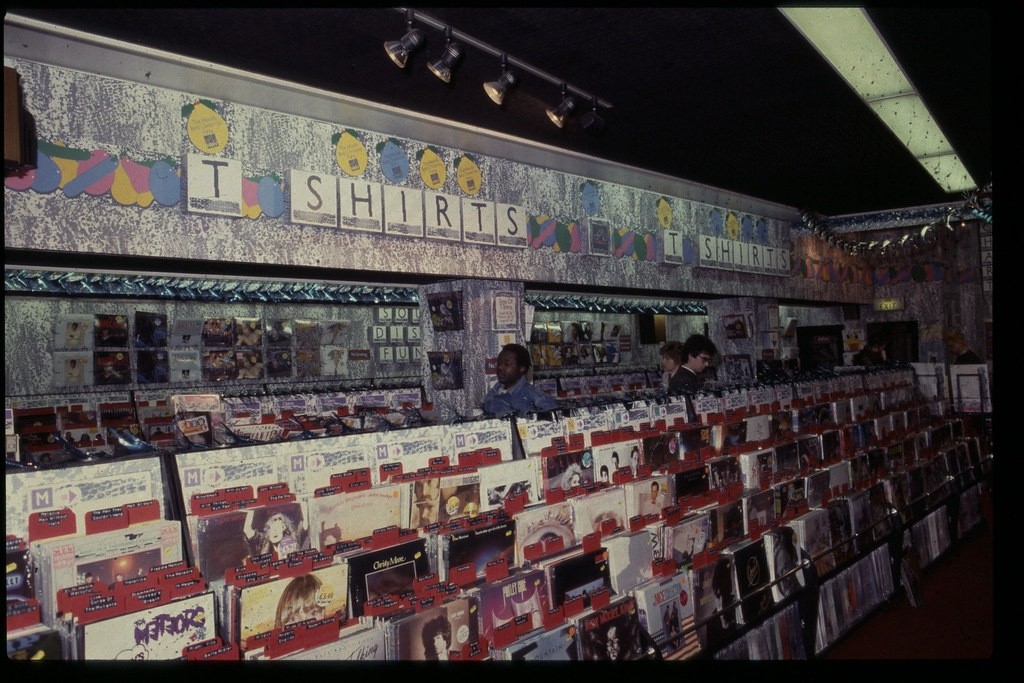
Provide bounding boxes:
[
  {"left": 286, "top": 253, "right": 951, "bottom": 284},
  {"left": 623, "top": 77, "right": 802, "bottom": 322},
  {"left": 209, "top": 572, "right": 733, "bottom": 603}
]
[{"left": 2, "top": 313, "right": 993, "bottom": 670}]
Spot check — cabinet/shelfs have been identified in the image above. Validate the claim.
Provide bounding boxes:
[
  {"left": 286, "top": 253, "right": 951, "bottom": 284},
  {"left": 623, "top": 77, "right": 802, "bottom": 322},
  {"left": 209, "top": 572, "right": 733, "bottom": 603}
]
[
  {"left": 490, "top": 384, "right": 817, "bottom": 660},
  {"left": 169, "top": 391, "right": 588, "bottom": 660},
  {"left": 4, "top": 400, "right": 227, "bottom": 661},
  {"left": 682, "top": 364, "right": 919, "bottom": 653},
  {"left": 787, "top": 363, "right": 990, "bottom": 615}
]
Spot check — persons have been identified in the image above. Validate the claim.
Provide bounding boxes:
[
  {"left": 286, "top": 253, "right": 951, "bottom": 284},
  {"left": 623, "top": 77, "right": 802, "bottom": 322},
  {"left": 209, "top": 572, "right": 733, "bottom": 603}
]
[
  {"left": 668, "top": 332, "right": 718, "bottom": 398},
  {"left": 484, "top": 343, "right": 559, "bottom": 416},
  {"left": 561, "top": 463, "right": 582, "bottom": 492},
  {"left": 599, "top": 464, "right": 609, "bottom": 483},
  {"left": 852, "top": 327, "right": 889, "bottom": 365},
  {"left": 711, "top": 466, "right": 724, "bottom": 489},
  {"left": 701, "top": 466, "right": 715, "bottom": 491},
  {"left": 413, "top": 479, "right": 432, "bottom": 502},
  {"left": 786, "top": 483, "right": 799, "bottom": 509},
  {"left": 671, "top": 601, "right": 680, "bottom": 632},
  {"left": 642, "top": 481, "right": 665, "bottom": 516},
  {"left": 630, "top": 445, "right": 641, "bottom": 479},
  {"left": 415, "top": 504, "right": 433, "bottom": 531},
  {"left": 274, "top": 574, "right": 327, "bottom": 630},
  {"left": 251, "top": 509, "right": 313, "bottom": 559},
  {"left": 85, "top": 573, "right": 92, "bottom": 584},
  {"left": 663, "top": 606, "right": 671, "bottom": 636},
  {"left": 114, "top": 574, "right": 124, "bottom": 581},
  {"left": 773, "top": 527, "right": 802, "bottom": 598},
  {"left": 659, "top": 341, "right": 686, "bottom": 396},
  {"left": 607, "top": 624, "right": 627, "bottom": 662},
  {"left": 65, "top": 360, "right": 81, "bottom": 385},
  {"left": 942, "top": 323, "right": 983, "bottom": 366},
  {"left": 236, "top": 353, "right": 263, "bottom": 379},
  {"left": 423, "top": 616, "right": 461, "bottom": 663},
  {"left": 582, "top": 590, "right": 591, "bottom": 608},
  {"left": 235, "top": 323, "right": 262, "bottom": 347},
  {"left": 650, "top": 432, "right": 681, "bottom": 466},
  {"left": 610, "top": 452, "right": 620, "bottom": 481},
  {"left": 66, "top": 321, "right": 82, "bottom": 347},
  {"left": 269, "top": 324, "right": 289, "bottom": 343},
  {"left": 711, "top": 554, "right": 737, "bottom": 626}
]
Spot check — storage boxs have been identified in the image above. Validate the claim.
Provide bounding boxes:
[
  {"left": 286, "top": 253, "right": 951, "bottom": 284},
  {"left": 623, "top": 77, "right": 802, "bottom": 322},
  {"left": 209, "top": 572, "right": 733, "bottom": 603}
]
[{"left": 7, "top": 367, "right": 995, "bottom": 659}]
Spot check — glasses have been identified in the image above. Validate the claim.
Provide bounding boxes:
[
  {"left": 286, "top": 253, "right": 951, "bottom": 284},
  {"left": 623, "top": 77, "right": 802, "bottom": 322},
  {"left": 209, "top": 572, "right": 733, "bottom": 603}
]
[{"left": 697, "top": 355, "right": 713, "bottom": 364}]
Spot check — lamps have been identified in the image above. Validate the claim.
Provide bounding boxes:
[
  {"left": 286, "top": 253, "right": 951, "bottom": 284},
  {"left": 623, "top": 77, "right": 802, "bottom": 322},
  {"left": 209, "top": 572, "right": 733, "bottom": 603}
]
[{"left": 383, "top": 8, "right": 616, "bottom": 135}]
